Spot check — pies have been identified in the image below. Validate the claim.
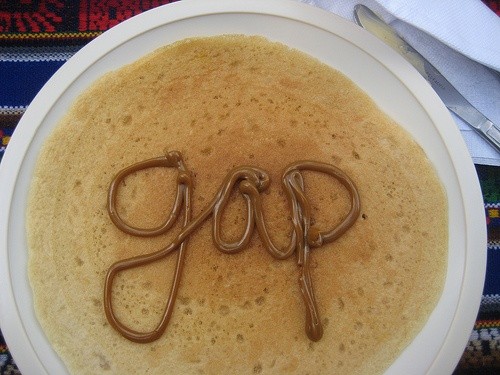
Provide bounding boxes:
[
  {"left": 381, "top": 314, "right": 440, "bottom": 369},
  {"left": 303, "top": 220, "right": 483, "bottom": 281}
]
[{"left": 28, "top": 33, "right": 449, "bottom": 375}]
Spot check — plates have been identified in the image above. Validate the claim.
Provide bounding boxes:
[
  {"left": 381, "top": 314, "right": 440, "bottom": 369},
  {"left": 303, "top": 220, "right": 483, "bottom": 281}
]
[{"left": 0, "top": 0, "right": 488, "bottom": 375}]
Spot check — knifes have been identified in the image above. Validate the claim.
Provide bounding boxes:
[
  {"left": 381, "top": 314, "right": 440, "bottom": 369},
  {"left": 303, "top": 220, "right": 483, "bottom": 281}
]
[{"left": 354, "top": 4, "right": 500, "bottom": 153}]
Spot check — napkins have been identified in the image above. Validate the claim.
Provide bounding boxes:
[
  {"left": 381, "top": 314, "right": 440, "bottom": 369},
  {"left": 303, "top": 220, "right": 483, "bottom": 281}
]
[{"left": 309, "top": 1, "right": 500, "bottom": 166}]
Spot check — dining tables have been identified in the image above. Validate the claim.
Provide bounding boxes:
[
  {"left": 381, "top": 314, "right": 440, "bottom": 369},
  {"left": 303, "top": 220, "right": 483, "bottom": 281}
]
[{"left": 0, "top": 0, "right": 500, "bottom": 375}]
